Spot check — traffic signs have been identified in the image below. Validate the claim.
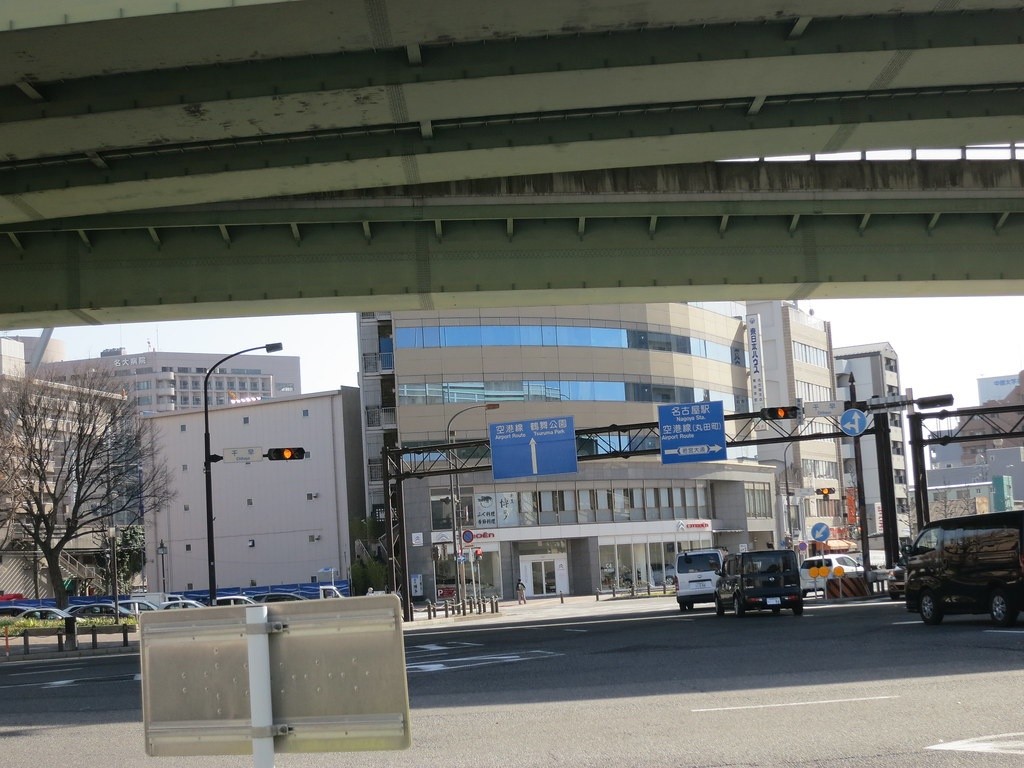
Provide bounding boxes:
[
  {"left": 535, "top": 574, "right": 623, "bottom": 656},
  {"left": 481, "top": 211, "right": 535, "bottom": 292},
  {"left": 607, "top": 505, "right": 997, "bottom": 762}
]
[
  {"left": 657, "top": 400, "right": 729, "bottom": 466},
  {"left": 490, "top": 417, "right": 580, "bottom": 481}
]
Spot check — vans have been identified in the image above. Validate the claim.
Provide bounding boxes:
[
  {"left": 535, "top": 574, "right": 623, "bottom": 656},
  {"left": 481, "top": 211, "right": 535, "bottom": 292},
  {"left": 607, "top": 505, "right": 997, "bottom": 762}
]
[
  {"left": 713, "top": 550, "right": 806, "bottom": 619},
  {"left": 888, "top": 509, "right": 1024, "bottom": 626}
]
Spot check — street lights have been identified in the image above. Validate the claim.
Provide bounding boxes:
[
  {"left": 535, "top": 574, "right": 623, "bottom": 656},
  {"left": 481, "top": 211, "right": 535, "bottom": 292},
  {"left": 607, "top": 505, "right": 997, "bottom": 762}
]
[
  {"left": 109, "top": 524, "right": 123, "bottom": 624},
  {"left": 156, "top": 539, "right": 169, "bottom": 594},
  {"left": 446, "top": 403, "right": 500, "bottom": 615},
  {"left": 202, "top": 343, "right": 283, "bottom": 607},
  {"left": 784, "top": 432, "right": 825, "bottom": 534}
]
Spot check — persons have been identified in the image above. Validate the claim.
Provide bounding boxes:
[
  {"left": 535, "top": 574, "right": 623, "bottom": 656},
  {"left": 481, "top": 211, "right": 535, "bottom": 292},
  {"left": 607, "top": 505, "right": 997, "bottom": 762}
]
[
  {"left": 366, "top": 588, "right": 373, "bottom": 596},
  {"left": 516, "top": 579, "right": 527, "bottom": 605}
]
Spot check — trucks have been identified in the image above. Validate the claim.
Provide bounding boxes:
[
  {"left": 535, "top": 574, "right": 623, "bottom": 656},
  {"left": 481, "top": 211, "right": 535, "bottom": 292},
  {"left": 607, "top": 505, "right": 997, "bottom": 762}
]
[{"left": 319, "top": 586, "right": 345, "bottom": 599}]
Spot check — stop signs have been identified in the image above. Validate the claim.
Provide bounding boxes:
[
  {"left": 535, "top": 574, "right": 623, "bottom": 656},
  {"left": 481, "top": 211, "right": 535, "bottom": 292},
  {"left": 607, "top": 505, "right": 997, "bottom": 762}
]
[{"left": 437, "top": 588, "right": 457, "bottom": 598}]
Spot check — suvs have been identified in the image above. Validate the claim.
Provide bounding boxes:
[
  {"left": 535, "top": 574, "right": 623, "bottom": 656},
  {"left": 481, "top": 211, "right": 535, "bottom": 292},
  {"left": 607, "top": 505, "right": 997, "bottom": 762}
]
[
  {"left": 623, "top": 563, "right": 676, "bottom": 587},
  {"left": 674, "top": 546, "right": 729, "bottom": 613},
  {"left": 800, "top": 554, "right": 865, "bottom": 598}
]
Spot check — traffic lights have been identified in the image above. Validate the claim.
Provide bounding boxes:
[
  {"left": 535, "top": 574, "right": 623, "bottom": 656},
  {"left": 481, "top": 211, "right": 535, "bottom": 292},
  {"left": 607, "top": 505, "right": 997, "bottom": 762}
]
[
  {"left": 815, "top": 488, "right": 836, "bottom": 495},
  {"left": 760, "top": 406, "right": 799, "bottom": 420},
  {"left": 268, "top": 447, "right": 306, "bottom": 461},
  {"left": 475, "top": 549, "right": 483, "bottom": 560}
]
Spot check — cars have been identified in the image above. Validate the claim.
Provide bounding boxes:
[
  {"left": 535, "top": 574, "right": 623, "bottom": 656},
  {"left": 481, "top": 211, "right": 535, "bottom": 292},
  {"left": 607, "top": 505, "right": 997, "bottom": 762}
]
[{"left": 0, "top": 592, "right": 310, "bottom": 622}]
